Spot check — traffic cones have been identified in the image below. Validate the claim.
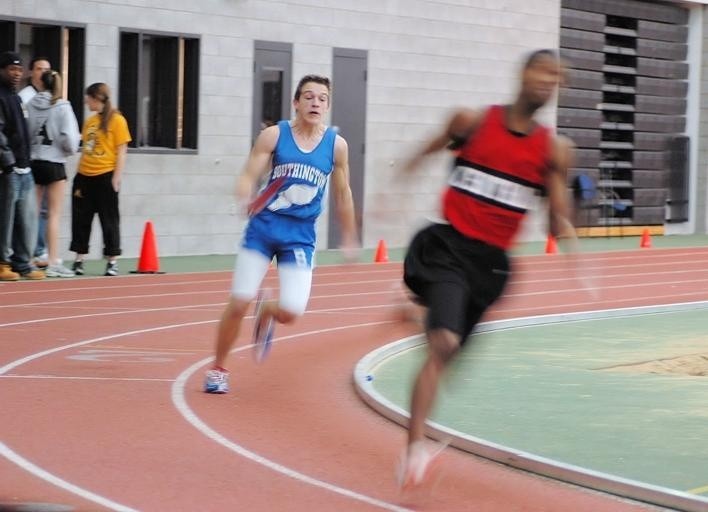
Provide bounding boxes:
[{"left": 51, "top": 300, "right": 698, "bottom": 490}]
[
  {"left": 374, "top": 239, "right": 390, "bottom": 263},
  {"left": 640, "top": 229, "right": 652, "bottom": 247},
  {"left": 131, "top": 221, "right": 166, "bottom": 273},
  {"left": 545, "top": 233, "right": 559, "bottom": 254}
]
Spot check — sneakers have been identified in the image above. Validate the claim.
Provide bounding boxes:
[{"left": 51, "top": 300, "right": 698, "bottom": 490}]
[
  {"left": 0, "top": 260, "right": 118, "bottom": 281},
  {"left": 204, "top": 369, "right": 229, "bottom": 393}
]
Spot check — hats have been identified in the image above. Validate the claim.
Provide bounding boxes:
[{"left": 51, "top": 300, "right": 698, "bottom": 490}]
[{"left": 2, "top": 51, "right": 24, "bottom": 65}]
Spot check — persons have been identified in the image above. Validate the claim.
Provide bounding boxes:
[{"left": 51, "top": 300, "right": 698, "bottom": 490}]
[
  {"left": 200, "top": 72, "right": 361, "bottom": 395},
  {"left": 1, "top": 50, "right": 133, "bottom": 283},
  {"left": 367, "top": 43, "right": 605, "bottom": 493}
]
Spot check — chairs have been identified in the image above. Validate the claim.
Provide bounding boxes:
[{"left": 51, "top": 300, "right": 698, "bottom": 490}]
[{"left": 577, "top": 174, "right": 626, "bottom": 239}]
